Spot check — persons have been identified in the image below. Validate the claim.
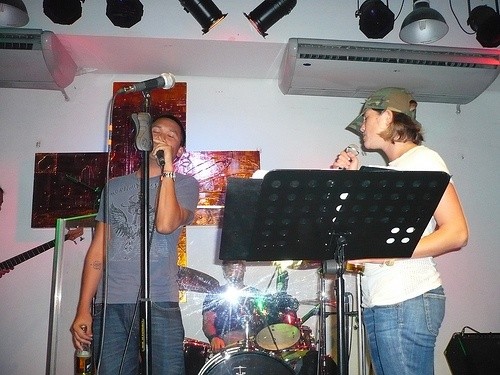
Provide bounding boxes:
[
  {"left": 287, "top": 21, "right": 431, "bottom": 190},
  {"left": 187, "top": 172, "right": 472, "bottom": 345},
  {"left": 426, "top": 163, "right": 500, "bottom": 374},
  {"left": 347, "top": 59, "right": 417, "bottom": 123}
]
[
  {"left": 202, "top": 262, "right": 262, "bottom": 351},
  {"left": 71, "top": 115, "right": 199, "bottom": 375},
  {"left": 331, "top": 87, "right": 469, "bottom": 375}
]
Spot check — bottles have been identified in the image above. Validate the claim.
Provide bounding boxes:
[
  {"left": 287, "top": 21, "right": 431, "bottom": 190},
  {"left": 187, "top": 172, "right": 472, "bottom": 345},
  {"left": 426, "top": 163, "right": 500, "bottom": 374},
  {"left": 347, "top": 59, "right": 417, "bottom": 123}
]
[{"left": 73, "top": 324, "right": 94, "bottom": 375}]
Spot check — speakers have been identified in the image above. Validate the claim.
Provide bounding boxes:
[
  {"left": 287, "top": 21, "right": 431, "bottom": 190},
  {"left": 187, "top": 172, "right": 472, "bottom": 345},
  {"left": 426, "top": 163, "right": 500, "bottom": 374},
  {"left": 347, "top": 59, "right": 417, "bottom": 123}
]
[{"left": 444, "top": 332, "right": 500, "bottom": 375}]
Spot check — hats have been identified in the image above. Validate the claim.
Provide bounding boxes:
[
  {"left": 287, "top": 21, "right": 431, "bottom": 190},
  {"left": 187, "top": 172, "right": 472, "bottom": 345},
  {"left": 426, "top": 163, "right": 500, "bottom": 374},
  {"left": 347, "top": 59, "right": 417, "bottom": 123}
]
[{"left": 345, "top": 87, "right": 418, "bottom": 137}]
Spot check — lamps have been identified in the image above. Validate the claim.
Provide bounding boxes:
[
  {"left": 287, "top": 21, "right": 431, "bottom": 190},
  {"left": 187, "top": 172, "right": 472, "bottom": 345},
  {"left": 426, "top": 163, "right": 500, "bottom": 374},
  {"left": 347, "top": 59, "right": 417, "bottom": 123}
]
[
  {"left": 179, "top": 0, "right": 228, "bottom": 35},
  {"left": 355, "top": 0, "right": 404, "bottom": 39},
  {"left": 450, "top": 0, "right": 500, "bottom": 48},
  {"left": 243, "top": 0, "right": 297, "bottom": 39},
  {"left": 0, "top": 0, "right": 29, "bottom": 28},
  {"left": 399, "top": 0, "right": 449, "bottom": 44},
  {"left": 105, "top": 0, "right": 143, "bottom": 28},
  {"left": 43, "top": 0, "right": 85, "bottom": 25}
]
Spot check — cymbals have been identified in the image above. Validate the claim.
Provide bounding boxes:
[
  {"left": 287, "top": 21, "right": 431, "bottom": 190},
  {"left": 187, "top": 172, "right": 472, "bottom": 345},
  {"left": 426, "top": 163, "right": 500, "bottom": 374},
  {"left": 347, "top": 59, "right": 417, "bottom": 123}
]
[
  {"left": 297, "top": 295, "right": 339, "bottom": 307},
  {"left": 177, "top": 264, "right": 219, "bottom": 291}
]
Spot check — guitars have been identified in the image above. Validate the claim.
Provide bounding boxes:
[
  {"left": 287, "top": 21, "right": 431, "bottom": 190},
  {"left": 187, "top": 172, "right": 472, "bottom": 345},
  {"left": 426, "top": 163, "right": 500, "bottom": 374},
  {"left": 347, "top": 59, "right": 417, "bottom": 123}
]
[{"left": 0, "top": 225, "right": 86, "bottom": 279}]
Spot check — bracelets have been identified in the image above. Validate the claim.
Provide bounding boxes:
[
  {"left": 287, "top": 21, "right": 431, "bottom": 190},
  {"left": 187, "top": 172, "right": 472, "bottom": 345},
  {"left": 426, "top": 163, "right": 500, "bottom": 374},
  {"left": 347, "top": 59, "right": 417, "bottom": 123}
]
[{"left": 208, "top": 334, "right": 216, "bottom": 341}]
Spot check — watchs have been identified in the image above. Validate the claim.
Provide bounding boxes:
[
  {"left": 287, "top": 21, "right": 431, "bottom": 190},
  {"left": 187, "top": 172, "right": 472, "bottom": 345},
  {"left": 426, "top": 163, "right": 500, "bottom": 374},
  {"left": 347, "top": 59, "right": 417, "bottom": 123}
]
[{"left": 162, "top": 171, "right": 176, "bottom": 181}]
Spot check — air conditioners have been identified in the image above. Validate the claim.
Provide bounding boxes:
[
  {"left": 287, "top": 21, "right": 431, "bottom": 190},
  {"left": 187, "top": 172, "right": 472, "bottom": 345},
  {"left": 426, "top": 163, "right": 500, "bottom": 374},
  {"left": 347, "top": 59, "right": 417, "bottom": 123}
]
[
  {"left": 0, "top": 28, "right": 77, "bottom": 91},
  {"left": 278, "top": 37, "right": 500, "bottom": 106}
]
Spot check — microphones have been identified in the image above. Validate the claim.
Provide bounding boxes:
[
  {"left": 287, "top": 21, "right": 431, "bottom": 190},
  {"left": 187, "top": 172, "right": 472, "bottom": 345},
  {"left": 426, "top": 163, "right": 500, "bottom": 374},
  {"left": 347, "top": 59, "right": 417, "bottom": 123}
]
[
  {"left": 337, "top": 144, "right": 359, "bottom": 169},
  {"left": 118, "top": 73, "right": 176, "bottom": 95},
  {"left": 156, "top": 150, "right": 165, "bottom": 166}
]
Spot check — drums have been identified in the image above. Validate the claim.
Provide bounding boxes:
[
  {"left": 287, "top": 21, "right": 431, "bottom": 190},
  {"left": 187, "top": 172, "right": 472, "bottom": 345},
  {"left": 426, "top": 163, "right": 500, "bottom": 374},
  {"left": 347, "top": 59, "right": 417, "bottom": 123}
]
[
  {"left": 278, "top": 327, "right": 313, "bottom": 363},
  {"left": 175, "top": 338, "right": 210, "bottom": 375},
  {"left": 255, "top": 307, "right": 300, "bottom": 353},
  {"left": 195, "top": 344, "right": 297, "bottom": 375}
]
